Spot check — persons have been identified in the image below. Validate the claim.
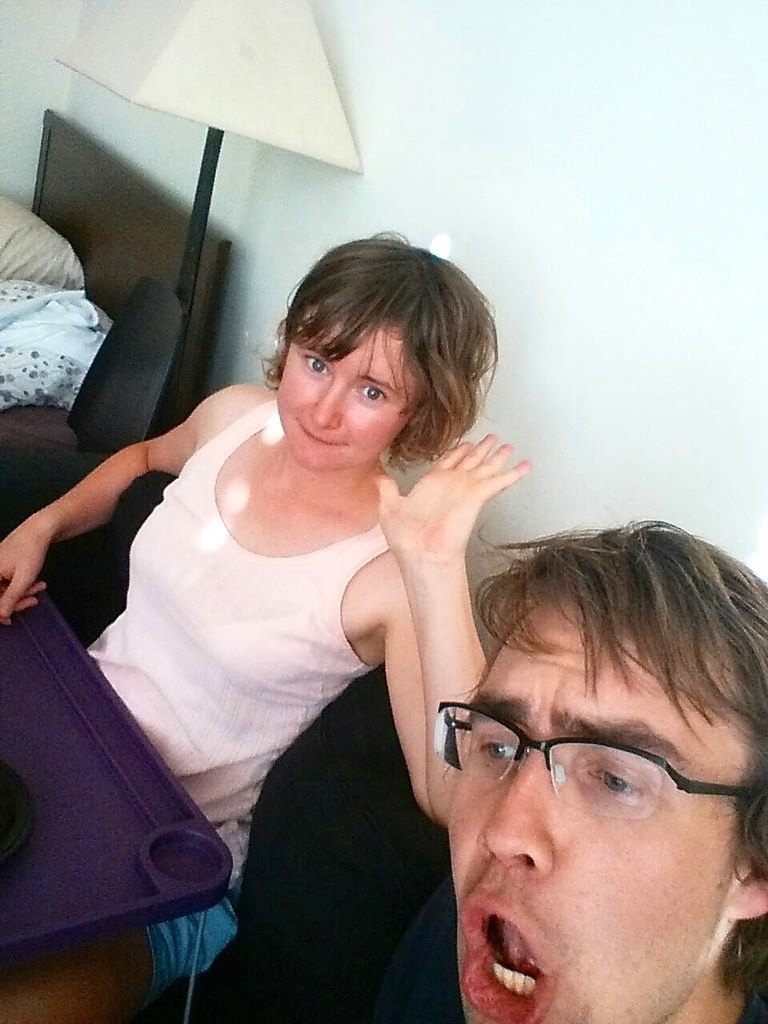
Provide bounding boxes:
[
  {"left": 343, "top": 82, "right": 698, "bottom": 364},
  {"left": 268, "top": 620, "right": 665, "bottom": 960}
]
[
  {"left": 1, "top": 239, "right": 535, "bottom": 1024},
  {"left": 431, "top": 521, "right": 768, "bottom": 1023}
]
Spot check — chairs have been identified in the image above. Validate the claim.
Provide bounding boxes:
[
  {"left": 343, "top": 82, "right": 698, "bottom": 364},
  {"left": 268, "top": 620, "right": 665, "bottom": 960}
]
[{"left": 0, "top": 469, "right": 453, "bottom": 1024}]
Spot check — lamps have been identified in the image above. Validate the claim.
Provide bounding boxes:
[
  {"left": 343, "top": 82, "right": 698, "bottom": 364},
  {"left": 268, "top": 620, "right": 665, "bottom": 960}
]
[{"left": 52, "top": 0, "right": 363, "bottom": 438}]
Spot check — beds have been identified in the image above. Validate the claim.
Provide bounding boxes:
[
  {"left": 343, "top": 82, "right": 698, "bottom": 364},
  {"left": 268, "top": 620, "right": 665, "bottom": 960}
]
[{"left": 1, "top": 105, "right": 234, "bottom": 526}]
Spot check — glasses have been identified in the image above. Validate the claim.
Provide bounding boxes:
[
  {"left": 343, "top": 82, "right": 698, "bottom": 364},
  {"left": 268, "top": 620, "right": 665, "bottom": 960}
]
[{"left": 433, "top": 701, "right": 748, "bottom": 821}]
